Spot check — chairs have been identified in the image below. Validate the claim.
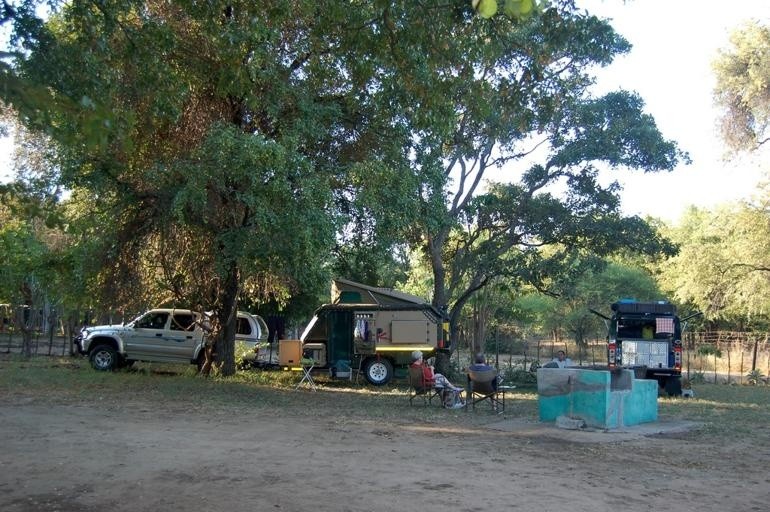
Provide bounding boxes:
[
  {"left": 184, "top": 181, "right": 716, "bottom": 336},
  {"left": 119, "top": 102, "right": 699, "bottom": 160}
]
[
  {"left": 465, "top": 367, "right": 508, "bottom": 419},
  {"left": 407, "top": 364, "right": 445, "bottom": 408}
]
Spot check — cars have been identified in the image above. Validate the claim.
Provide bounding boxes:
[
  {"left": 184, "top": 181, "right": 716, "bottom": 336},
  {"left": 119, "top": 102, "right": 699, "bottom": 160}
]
[
  {"left": 73, "top": 308, "right": 269, "bottom": 374},
  {"left": 0, "top": 303, "right": 43, "bottom": 329}
]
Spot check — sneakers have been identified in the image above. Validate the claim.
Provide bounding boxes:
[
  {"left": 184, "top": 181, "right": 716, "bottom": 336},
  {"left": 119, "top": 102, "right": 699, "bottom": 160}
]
[{"left": 453, "top": 388, "right": 464, "bottom": 395}]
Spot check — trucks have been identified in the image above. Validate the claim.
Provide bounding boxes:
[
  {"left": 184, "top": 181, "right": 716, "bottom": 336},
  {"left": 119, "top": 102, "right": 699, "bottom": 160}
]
[{"left": 606, "top": 298, "right": 681, "bottom": 397}]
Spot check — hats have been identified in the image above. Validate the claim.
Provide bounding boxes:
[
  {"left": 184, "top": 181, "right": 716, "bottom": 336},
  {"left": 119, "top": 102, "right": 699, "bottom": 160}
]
[{"left": 411, "top": 351, "right": 423, "bottom": 361}]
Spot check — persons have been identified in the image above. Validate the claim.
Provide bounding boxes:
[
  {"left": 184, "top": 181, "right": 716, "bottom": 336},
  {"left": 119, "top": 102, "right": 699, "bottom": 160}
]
[
  {"left": 529, "top": 349, "right": 567, "bottom": 378},
  {"left": 466, "top": 353, "right": 497, "bottom": 394},
  {"left": 411, "top": 350, "right": 463, "bottom": 408}
]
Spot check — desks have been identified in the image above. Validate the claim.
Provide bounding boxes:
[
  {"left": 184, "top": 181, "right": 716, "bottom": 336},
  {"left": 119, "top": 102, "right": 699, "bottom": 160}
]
[{"left": 295, "top": 364, "right": 318, "bottom": 392}]
